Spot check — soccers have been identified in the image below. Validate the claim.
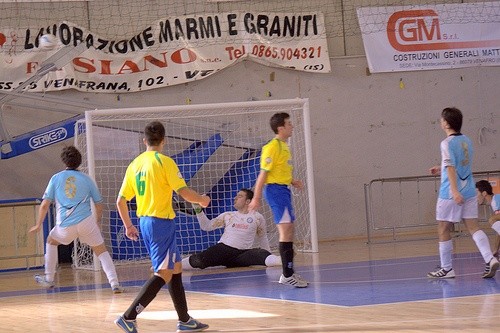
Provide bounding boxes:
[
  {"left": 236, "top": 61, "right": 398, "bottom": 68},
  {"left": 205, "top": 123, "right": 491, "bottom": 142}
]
[{"left": 40, "top": 34, "right": 57, "bottom": 51}]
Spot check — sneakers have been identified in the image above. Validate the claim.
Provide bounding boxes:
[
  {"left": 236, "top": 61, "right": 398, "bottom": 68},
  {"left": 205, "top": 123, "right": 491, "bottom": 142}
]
[
  {"left": 33, "top": 274, "right": 55, "bottom": 288},
  {"left": 114, "top": 313, "right": 138, "bottom": 333},
  {"left": 112, "top": 285, "right": 125, "bottom": 293},
  {"left": 427, "top": 265, "right": 456, "bottom": 278},
  {"left": 278, "top": 274, "right": 310, "bottom": 288},
  {"left": 481, "top": 257, "right": 499, "bottom": 278},
  {"left": 177, "top": 316, "right": 209, "bottom": 333}
]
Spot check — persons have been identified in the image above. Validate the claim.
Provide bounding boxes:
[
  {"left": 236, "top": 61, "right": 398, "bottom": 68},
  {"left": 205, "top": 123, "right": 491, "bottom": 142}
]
[
  {"left": 149, "top": 188, "right": 298, "bottom": 273},
  {"left": 427, "top": 106, "right": 500, "bottom": 279},
  {"left": 116, "top": 122, "right": 211, "bottom": 333},
  {"left": 29, "top": 146, "right": 126, "bottom": 294},
  {"left": 247, "top": 113, "right": 310, "bottom": 288}
]
